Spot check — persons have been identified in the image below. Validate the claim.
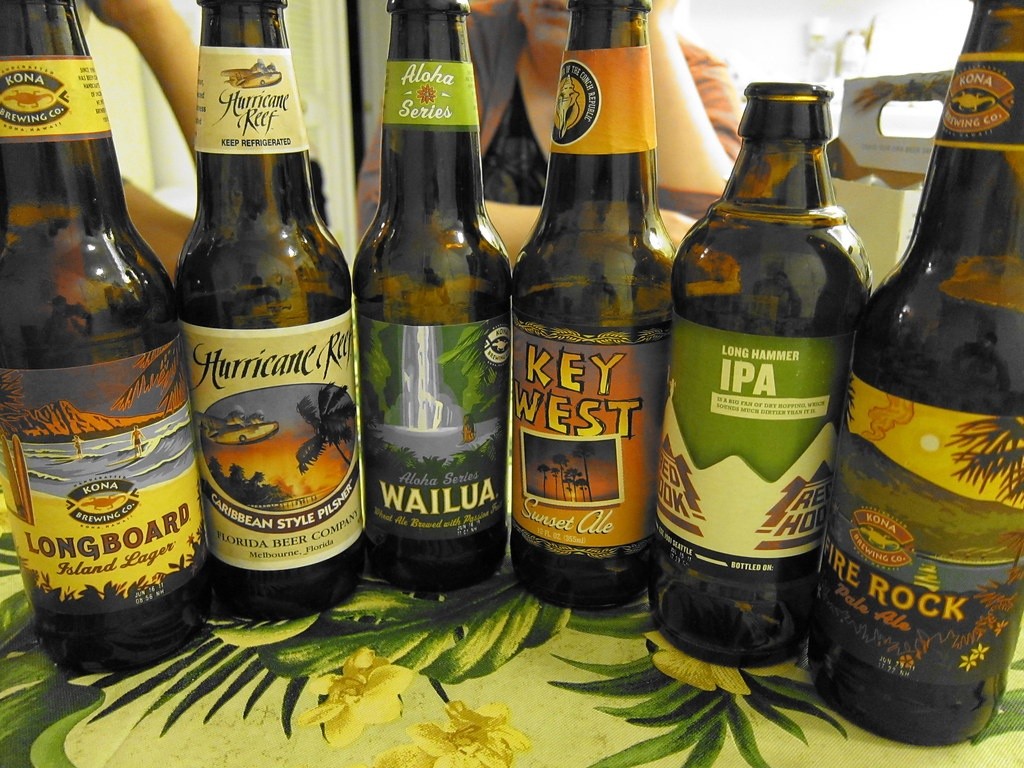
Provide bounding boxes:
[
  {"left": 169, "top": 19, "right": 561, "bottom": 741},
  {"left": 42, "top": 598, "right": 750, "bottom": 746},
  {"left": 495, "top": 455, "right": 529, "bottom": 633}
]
[
  {"left": 0, "top": 4, "right": 321, "bottom": 282},
  {"left": 360, "top": 1, "right": 747, "bottom": 263}
]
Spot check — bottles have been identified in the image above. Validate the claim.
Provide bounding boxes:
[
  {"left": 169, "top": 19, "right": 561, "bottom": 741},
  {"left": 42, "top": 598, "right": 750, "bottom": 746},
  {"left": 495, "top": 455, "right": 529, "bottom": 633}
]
[
  {"left": 650, "top": 80, "right": 876, "bottom": 661},
  {"left": 353, "top": 0, "right": 510, "bottom": 588},
  {"left": 172, "top": 2, "right": 369, "bottom": 620},
  {"left": 499, "top": 0, "right": 680, "bottom": 612},
  {"left": 0, "top": 0, "right": 208, "bottom": 671},
  {"left": 806, "top": 0, "right": 1024, "bottom": 748}
]
